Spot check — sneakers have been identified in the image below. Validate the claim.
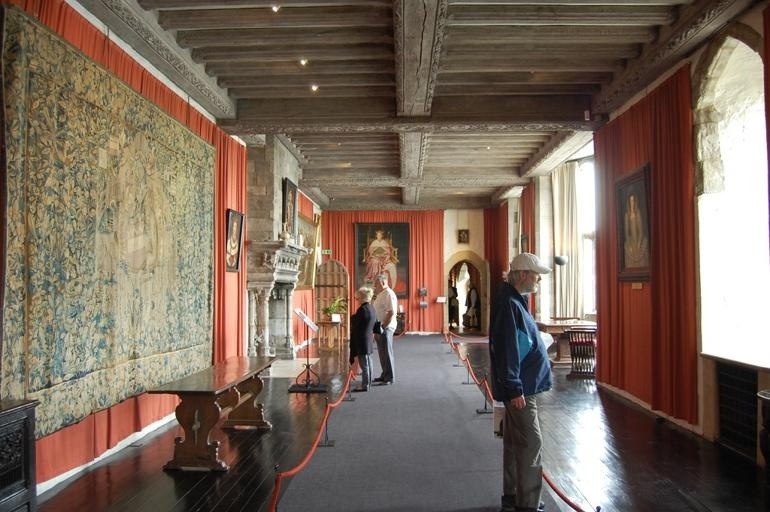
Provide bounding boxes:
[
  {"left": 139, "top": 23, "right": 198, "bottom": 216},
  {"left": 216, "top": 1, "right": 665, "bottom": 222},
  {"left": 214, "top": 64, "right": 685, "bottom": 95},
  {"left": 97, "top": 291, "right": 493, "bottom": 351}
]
[
  {"left": 501, "top": 494, "right": 545, "bottom": 511},
  {"left": 353, "top": 387, "right": 367, "bottom": 392}
]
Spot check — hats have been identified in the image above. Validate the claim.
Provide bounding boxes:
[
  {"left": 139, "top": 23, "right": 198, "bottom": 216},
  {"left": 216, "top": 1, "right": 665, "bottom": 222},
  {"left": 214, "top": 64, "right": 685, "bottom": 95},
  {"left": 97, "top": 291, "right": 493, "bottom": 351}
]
[{"left": 510, "top": 252, "right": 552, "bottom": 275}]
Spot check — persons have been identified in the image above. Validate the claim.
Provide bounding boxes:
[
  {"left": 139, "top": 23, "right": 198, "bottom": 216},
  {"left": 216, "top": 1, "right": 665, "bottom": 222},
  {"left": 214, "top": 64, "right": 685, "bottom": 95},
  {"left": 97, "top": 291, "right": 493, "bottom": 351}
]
[
  {"left": 371, "top": 276, "right": 397, "bottom": 384},
  {"left": 489, "top": 252, "right": 553, "bottom": 512},
  {"left": 463, "top": 283, "right": 479, "bottom": 330},
  {"left": 623, "top": 193, "right": 650, "bottom": 268},
  {"left": 448, "top": 281, "right": 459, "bottom": 329},
  {"left": 288, "top": 189, "right": 294, "bottom": 223},
  {"left": 367, "top": 230, "right": 390, "bottom": 283},
  {"left": 352, "top": 287, "right": 376, "bottom": 391},
  {"left": 227, "top": 216, "right": 239, "bottom": 267}
]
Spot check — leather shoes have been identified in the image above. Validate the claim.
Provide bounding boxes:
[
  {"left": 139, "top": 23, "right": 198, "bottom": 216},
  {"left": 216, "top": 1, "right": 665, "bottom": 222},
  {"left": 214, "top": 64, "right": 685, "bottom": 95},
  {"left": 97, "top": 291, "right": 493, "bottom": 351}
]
[{"left": 375, "top": 378, "right": 391, "bottom": 386}]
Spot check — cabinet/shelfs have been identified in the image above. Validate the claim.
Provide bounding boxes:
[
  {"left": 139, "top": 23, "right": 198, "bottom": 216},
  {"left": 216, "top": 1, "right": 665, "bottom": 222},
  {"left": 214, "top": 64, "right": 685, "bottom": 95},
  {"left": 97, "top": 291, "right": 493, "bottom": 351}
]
[{"left": 0, "top": 397, "right": 42, "bottom": 512}]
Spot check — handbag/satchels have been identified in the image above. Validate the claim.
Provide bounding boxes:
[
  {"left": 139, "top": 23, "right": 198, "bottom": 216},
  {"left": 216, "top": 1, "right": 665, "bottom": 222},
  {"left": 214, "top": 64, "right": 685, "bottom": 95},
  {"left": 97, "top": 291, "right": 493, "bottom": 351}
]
[
  {"left": 451, "top": 298, "right": 458, "bottom": 307},
  {"left": 462, "top": 314, "right": 478, "bottom": 327},
  {"left": 373, "top": 322, "right": 384, "bottom": 335}
]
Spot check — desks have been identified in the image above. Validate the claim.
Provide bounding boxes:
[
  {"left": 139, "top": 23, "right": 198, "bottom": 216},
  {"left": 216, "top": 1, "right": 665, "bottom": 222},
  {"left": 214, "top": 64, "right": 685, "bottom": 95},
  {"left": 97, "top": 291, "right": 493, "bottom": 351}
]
[
  {"left": 146, "top": 352, "right": 278, "bottom": 476},
  {"left": 536, "top": 316, "right": 597, "bottom": 361}
]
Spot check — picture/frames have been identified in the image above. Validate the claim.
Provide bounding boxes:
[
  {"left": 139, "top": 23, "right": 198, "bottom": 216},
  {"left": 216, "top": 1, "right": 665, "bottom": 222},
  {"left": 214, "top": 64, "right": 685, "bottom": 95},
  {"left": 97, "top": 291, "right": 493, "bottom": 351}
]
[
  {"left": 224, "top": 207, "right": 247, "bottom": 274},
  {"left": 294, "top": 211, "right": 319, "bottom": 293},
  {"left": 355, "top": 223, "right": 410, "bottom": 300},
  {"left": 281, "top": 177, "right": 298, "bottom": 237},
  {"left": 612, "top": 161, "right": 657, "bottom": 284}
]
